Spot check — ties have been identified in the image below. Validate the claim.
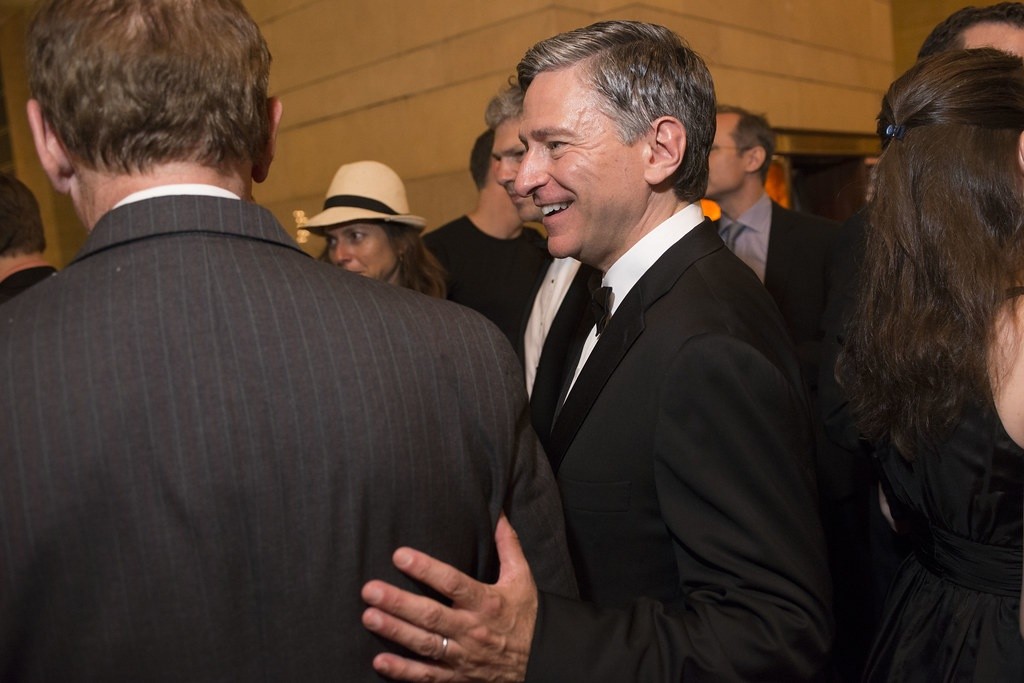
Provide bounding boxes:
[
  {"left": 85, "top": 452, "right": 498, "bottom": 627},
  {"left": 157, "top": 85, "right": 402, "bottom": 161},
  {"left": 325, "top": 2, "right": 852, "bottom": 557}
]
[{"left": 725, "top": 223, "right": 746, "bottom": 251}]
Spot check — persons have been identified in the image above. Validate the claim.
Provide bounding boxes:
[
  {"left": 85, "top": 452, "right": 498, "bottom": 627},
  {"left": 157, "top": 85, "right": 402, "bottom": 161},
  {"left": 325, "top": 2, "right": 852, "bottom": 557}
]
[
  {"left": 294, "top": 160, "right": 447, "bottom": 299},
  {"left": 484, "top": 81, "right": 602, "bottom": 440},
  {"left": 917, "top": 1, "right": 1024, "bottom": 64},
  {"left": 422, "top": 126, "right": 545, "bottom": 357},
  {"left": 0, "top": 172, "right": 59, "bottom": 302},
  {"left": 705, "top": 104, "right": 861, "bottom": 325},
  {"left": 842, "top": 49, "right": 1024, "bottom": 679},
  {"left": 341, "top": 23, "right": 840, "bottom": 683},
  {"left": 0, "top": 0, "right": 582, "bottom": 682}
]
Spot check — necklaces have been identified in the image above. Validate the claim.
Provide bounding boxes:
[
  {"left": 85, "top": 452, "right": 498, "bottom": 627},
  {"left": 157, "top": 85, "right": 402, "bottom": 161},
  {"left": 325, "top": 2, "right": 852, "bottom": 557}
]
[{"left": 0, "top": 260, "right": 46, "bottom": 279}]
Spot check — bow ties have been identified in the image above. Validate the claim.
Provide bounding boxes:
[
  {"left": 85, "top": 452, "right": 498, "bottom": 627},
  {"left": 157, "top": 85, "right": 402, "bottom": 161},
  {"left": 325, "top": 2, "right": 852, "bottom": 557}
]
[{"left": 587, "top": 274, "right": 612, "bottom": 336}]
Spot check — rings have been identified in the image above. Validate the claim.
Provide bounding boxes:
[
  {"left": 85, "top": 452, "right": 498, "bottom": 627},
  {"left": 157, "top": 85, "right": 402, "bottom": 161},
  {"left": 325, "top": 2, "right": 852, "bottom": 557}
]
[{"left": 434, "top": 638, "right": 447, "bottom": 660}]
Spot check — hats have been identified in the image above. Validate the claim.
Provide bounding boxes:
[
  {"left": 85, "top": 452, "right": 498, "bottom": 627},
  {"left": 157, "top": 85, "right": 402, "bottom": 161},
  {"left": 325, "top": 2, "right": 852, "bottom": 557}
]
[{"left": 297, "top": 160, "right": 428, "bottom": 233}]
